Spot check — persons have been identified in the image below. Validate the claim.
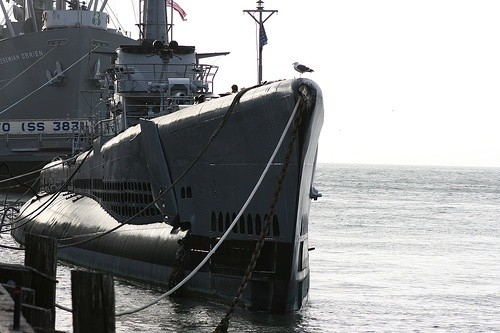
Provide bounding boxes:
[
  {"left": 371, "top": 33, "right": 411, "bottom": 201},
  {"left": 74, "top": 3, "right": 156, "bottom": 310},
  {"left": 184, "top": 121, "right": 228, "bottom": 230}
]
[
  {"left": 231, "top": 85, "right": 239, "bottom": 93},
  {"left": 199, "top": 94, "right": 205, "bottom": 102}
]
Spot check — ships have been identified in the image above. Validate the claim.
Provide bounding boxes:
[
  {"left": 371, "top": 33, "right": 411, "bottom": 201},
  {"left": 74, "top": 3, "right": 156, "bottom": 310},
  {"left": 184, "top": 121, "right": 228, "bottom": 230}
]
[
  {"left": 10, "top": 0, "right": 326, "bottom": 314},
  {"left": 0, "top": 0, "right": 229, "bottom": 192}
]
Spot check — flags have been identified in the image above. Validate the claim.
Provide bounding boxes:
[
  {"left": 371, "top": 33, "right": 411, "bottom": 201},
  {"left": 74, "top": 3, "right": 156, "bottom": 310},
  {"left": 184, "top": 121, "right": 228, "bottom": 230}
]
[
  {"left": 259, "top": 21, "right": 267, "bottom": 47},
  {"left": 166, "top": 0, "right": 187, "bottom": 22}
]
